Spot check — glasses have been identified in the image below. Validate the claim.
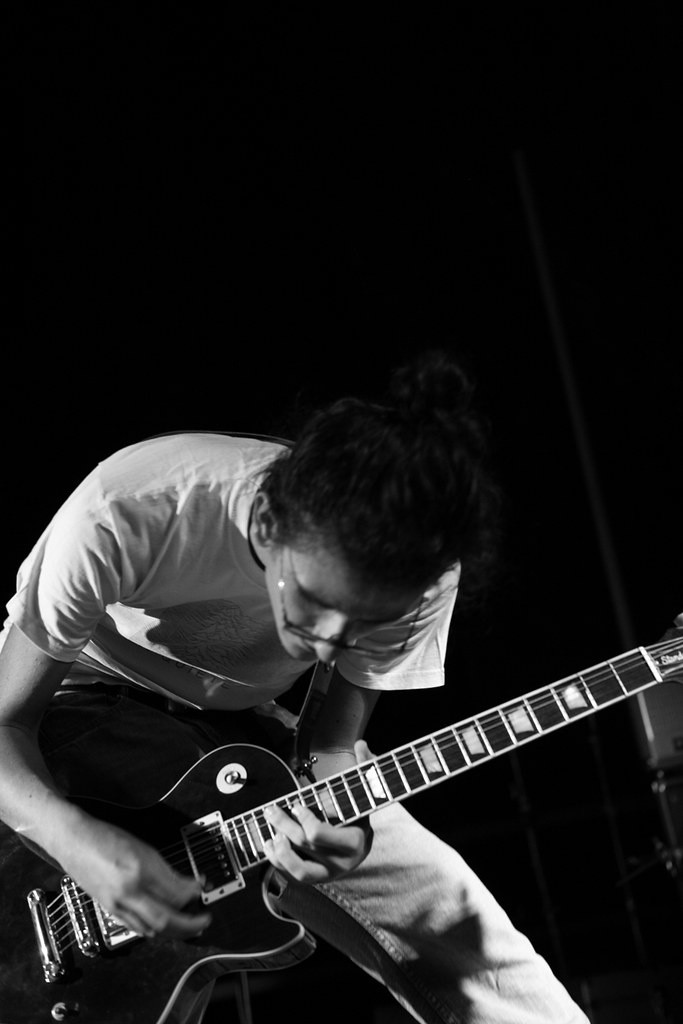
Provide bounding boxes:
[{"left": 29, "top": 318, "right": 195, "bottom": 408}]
[{"left": 280, "top": 542, "right": 424, "bottom": 661}]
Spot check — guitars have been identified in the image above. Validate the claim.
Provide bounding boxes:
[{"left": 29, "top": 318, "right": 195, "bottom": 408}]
[{"left": 0, "top": 633, "right": 683, "bottom": 1024}]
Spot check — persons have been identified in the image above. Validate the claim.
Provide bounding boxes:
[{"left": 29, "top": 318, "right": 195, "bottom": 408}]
[{"left": 0, "top": 401, "right": 591, "bottom": 1024}]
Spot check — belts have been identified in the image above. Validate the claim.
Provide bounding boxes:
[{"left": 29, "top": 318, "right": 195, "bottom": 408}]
[{"left": 58, "top": 681, "right": 202, "bottom": 718}]
